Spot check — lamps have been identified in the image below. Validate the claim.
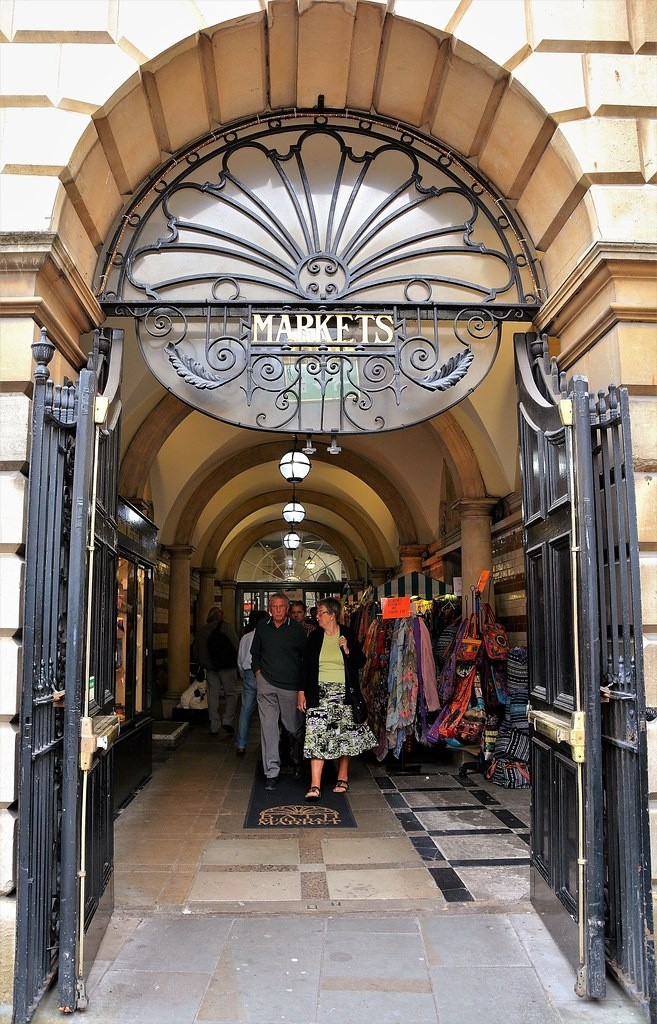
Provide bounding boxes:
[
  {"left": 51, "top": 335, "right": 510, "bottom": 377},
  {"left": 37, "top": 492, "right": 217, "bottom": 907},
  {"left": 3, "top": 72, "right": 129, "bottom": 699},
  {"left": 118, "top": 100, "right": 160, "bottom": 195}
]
[
  {"left": 284, "top": 552, "right": 296, "bottom": 578},
  {"left": 281, "top": 484, "right": 306, "bottom": 525},
  {"left": 278, "top": 434, "right": 311, "bottom": 484},
  {"left": 282, "top": 525, "right": 302, "bottom": 550},
  {"left": 304, "top": 551, "right": 316, "bottom": 570}
]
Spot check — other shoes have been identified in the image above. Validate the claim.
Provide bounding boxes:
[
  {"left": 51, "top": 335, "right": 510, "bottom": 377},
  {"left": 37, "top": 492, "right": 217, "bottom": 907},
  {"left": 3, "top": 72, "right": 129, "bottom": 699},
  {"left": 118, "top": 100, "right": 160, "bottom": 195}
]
[
  {"left": 223, "top": 724, "right": 235, "bottom": 733},
  {"left": 210, "top": 729, "right": 220, "bottom": 736},
  {"left": 294, "top": 761, "right": 308, "bottom": 779},
  {"left": 280, "top": 767, "right": 293, "bottom": 774},
  {"left": 264, "top": 776, "right": 280, "bottom": 790},
  {"left": 237, "top": 747, "right": 246, "bottom": 754}
]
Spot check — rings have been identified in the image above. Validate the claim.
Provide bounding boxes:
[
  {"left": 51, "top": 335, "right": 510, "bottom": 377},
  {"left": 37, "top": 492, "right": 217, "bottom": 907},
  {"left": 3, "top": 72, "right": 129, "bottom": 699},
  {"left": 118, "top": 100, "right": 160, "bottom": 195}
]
[{"left": 297, "top": 706, "right": 298, "bottom": 708}]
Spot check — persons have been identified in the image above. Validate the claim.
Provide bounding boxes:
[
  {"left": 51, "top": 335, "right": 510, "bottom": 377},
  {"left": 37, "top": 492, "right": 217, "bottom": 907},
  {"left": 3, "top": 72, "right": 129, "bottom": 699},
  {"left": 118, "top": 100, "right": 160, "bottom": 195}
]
[
  {"left": 193, "top": 607, "right": 239, "bottom": 737},
  {"left": 297, "top": 597, "right": 379, "bottom": 800},
  {"left": 237, "top": 610, "right": 270, "bottom": 754},
  {"left": 250, "top": 593, "right": 307, "bottom": 790},
  {"left": 290, "top": 601, "right": 318, "bottom": 636}
]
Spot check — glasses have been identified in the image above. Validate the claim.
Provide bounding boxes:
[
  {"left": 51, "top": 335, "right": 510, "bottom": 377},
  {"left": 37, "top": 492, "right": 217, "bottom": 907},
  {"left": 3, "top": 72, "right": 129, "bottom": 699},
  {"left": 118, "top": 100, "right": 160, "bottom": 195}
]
[{"left": 316, "top": 611, "right": 329, "bottom": 616}]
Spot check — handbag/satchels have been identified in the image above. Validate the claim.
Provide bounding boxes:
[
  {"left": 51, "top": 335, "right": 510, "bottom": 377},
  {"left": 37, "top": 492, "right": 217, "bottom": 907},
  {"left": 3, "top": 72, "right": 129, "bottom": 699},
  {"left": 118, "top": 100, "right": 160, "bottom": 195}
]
[
  {"left": 208, "top": 620, "right": 239, "bottom": 670},
  {"left": 432, "top": 603, "right": 530, "bottom": 789}
]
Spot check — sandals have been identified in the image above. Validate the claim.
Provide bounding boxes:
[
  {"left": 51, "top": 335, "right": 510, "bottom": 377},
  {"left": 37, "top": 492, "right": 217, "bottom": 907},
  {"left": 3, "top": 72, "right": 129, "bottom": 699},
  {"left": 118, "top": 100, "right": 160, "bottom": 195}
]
[
  {"left": 333, "top": 780, "right": 349, "bottom": 793},
  {"left": 304, "top": 787, "right": 322, "bottom": 798}
]
